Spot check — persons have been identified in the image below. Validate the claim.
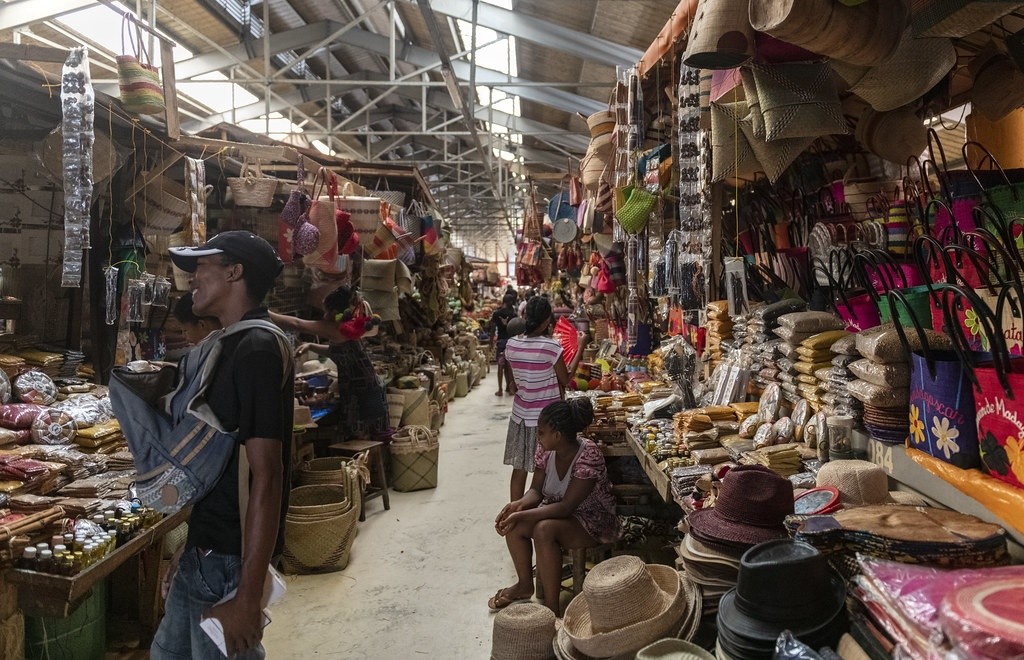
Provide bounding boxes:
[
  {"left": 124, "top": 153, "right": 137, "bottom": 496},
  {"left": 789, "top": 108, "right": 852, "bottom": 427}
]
[
  {"left": 268, "top": 286, "right": 393, "bottom": 445},
  {"left": 129, "top": 229, "right": 294, "bottom": 659},
  {"left": 172, "top": 292, "right": 223, "bottom": 346},
  {"left": 466, "top": 284, "right": 542, "bottom": 397},
  {"left": 488, "top": 396, "right": 619, "bottom": 617},
  {"left": 505, "top": 296, "right": 588, "bottom": 502}
]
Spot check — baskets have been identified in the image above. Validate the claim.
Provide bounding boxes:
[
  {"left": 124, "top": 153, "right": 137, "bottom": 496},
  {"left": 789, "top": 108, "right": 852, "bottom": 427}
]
[
  {"left": 122, "top": 173, "right": 188, "bottom": 238},
  {"left": 281, "top": 456, "right": 364, "bottom": 575}
]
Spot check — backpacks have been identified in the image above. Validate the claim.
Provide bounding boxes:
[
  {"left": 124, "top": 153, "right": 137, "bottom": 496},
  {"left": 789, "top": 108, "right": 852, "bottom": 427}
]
[{"left": 109, "top": 320, "right": 295, "bottom": 513}]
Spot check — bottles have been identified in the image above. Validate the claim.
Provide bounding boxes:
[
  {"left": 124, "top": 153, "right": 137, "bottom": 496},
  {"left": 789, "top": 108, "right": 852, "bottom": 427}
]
[{"left": 18, "top": 501, "right": 164, "bottom": 577}]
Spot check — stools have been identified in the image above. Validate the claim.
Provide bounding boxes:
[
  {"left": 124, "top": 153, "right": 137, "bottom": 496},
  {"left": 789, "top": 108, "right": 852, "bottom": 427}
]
[{"left": 536, "top": 543, "right": 610, "bottom": 599}]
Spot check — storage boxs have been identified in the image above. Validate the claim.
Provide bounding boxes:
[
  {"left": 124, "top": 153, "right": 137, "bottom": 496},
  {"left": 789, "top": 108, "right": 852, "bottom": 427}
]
[
  {"left": 127, "top": 172, "right": 420, "bottom": 264},
  {"left": 117, "top": 11, "right": 164, "bottom": 115},
  {"left": 576, "top": 84, "right": 618, "bottom": 191},
  {"left": 280, "top": 361, "right": 468, "bottom": 577}
]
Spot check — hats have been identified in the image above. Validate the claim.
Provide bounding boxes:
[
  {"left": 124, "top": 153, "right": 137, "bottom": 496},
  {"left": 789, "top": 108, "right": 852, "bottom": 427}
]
[
  {"left": 489, "top": 458, "right": 930, "bottom": 660},
  {"left": 168, "top": 231, "right": 283, "bottom": 288},
  {"left": 643, "top": 118, "right": 672, "bottom": 148}
]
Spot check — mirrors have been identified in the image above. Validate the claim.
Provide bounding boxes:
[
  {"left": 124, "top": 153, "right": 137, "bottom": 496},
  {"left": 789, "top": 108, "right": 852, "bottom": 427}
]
[{"left": 794, "top": 486, "right": 843, "bottom": 515}]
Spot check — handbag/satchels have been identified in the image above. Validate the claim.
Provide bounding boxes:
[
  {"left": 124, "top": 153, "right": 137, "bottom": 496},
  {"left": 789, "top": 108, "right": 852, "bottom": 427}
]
[
  {"left": 391, "top": 425, "right": 439, "bottom": 493},
  {"left": 513, "top": 176, "right": 658, "bottom": 286},
  {"left": 733, "top": 132, "right": 1023, "bottom": 489},
  {"left": 117, "top": 12, "right": 166, "bottom": 116},
  {"left": 579, "top": 88, "right": 619, "bottom": 188},
  {"left": 230, "top": 177, "right": 440, "bottom": 267}
]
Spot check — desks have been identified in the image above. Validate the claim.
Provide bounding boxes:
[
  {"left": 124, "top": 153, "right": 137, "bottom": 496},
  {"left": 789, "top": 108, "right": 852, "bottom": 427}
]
[
  {"left": 3, "top": 428, "right": 308, "bottom": 650},
  {"left": 585, "top": 439, "right": 684, "bottom": 517}
]
